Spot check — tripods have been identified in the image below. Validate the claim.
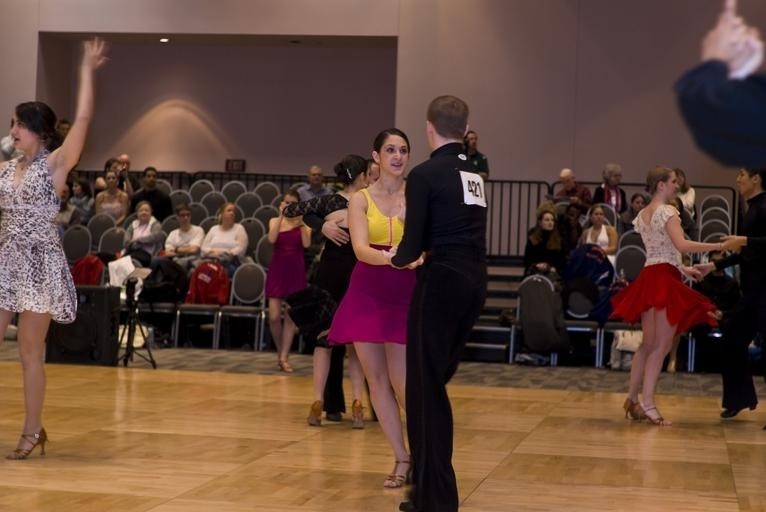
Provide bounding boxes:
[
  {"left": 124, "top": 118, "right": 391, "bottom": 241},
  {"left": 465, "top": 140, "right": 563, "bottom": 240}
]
[{"left": 119, "top": 309, "right": 157, "bottom": 368}]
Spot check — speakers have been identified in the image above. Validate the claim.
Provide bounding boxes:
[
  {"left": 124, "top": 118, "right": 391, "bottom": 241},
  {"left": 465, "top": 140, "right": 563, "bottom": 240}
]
[{"left": 44, "top": 284, "right": 121, "bottom": 366}]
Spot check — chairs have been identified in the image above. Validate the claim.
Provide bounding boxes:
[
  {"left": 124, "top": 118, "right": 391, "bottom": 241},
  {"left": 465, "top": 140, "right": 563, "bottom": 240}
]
[
  {"left": 508, "top": 195, "right": 732, "bottom": 373},
  {"left": 62, "top": 179, "right": 308, "bottom": 353}
]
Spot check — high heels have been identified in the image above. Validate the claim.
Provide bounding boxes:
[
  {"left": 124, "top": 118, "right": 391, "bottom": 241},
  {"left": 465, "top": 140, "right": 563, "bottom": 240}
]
[
  {"left": 306, "top": 401, "right": 324, "bottom": 427},
  {"left": 383, "top": 454, "right": 413, "bottom": 488},
  {"left": 720, "top": 404, "right": 756, "bottom": 417},
  {"left": 623, "top": 397, "right": 668, "bottom": 425},
  {"left": 352, "top": 399, "right": 367, "bottom": 429},
  {"left": 5, "top": 427, "right": 47, "bottom": 460},
  {"left": 277, "top": 355, "right": 293, "bottom": 372}
]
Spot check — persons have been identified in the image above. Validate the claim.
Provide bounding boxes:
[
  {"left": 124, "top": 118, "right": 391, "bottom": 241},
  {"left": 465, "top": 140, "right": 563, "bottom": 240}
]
[
  {"left": 164, "top": 202, "right": 249, "bottom": 280},
  {"left": 282, "top": 154, "right": 370, "bottom": 429},
  {"left": 382, "top": 94, "right": 488, "bottom": 512},
  {"left": 693, "top": 167, "right": 766, "bottom": 417},
  {"left": 465, "top": 131, "right": 489, "bottom": 180},
  {"left": 327, "top": 128, "right": 426, "bottom": 489},
  {"left": 268, "top": 164, "right": 328, "bottom": 372},
  {"left": 668, "top": 166, "right": 741, "bottom": 334},
  {"left": 104, "top": 165, "right": 173, "bottom": 268},
  {"left": 522, "top": 163, "right": 650, "bottom": 369},
  {"left": 609, "top": 165, "right": 742, "bottom": 425},
  {"left": 303, "top": 158, "right": 380, "bottom": 423},
  {"left": 0, "top": 37, "right": 109, "bottom": 460},
  {"left": 0, "top": 116, "right": 24, "bottom": 160},
  {"left": 49, "top": 119, "right": 134, "bottom": 237},
  {"left": 674, "top": 0, "right": 766, "bottom": 172}
]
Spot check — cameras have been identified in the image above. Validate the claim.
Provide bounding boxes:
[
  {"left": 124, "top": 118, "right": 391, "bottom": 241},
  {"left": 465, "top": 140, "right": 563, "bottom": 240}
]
[{"left": 121, "top": 166, "right": 126, "bottom": 173}]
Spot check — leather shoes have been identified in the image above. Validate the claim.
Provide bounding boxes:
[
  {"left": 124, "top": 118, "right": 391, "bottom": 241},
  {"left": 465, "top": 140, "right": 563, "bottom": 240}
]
[
  {"left": 398, "top": 491, "right": 415, "bottom": 511},
  {"left": 326, "top": 411, "right": 342, "bottom": 421}
]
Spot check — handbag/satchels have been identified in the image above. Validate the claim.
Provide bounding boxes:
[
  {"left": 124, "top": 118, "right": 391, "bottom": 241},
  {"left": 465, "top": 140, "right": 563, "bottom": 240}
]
[{"left": 72, "top": 248, "right": 230, "bottom": 305}]
